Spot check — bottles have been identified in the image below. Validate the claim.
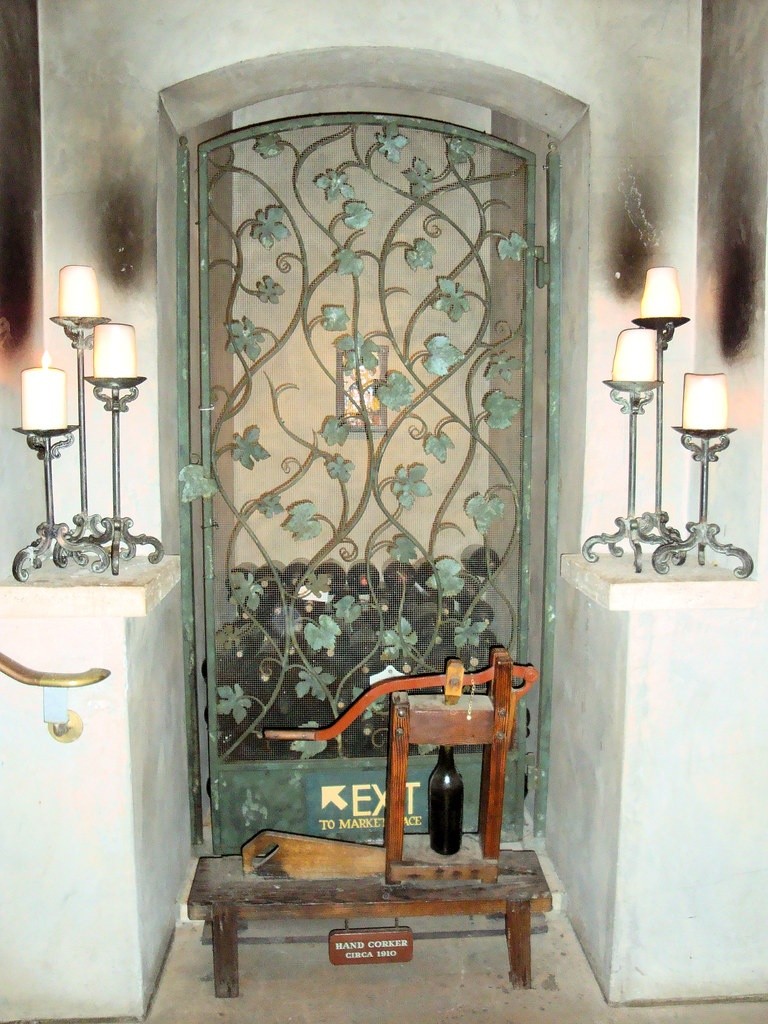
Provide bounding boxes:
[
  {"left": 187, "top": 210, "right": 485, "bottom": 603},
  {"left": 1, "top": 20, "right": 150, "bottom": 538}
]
[{"left": 430, "top": 746, "right": 464, "bottom": 854}]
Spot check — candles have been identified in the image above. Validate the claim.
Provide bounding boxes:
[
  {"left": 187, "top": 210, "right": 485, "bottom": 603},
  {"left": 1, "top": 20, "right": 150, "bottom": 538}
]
[
  {"left": 59, "top": 265, "right": 101, "bottom": 317},
  {"left": 20, "top": 350, "right": 68, "bottom": 430},
  {"left": 640, "top": 266, "right": 681, "bottom": 318},
  {"left": 613, "top": 329, "right": 658, "bottom": 382},
  {"left": 94, "top": 324, "right": 137, "bottom": 378},
  {"left": 682, "top": 372, "right": 729, "bottom": 430}
]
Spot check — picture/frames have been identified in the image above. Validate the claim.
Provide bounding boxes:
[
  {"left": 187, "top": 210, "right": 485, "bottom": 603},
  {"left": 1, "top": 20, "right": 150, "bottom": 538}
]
[{"left": 336, "top": 346, "right": 388, "bottom": 432}]
